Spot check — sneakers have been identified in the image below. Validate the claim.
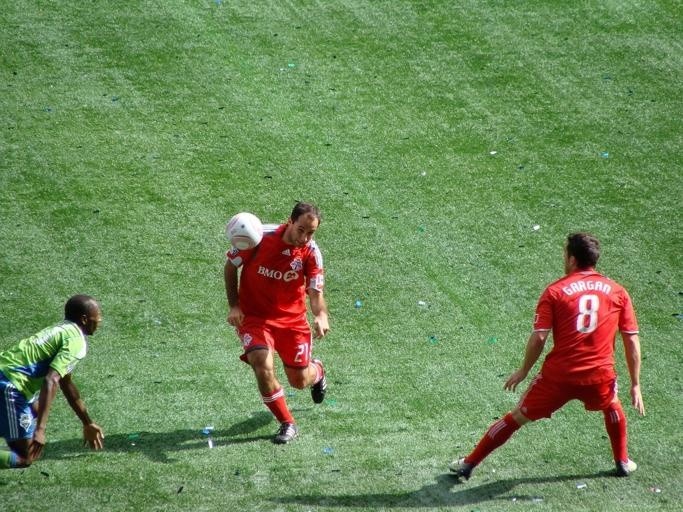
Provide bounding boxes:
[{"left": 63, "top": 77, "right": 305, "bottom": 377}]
[
  {"left": 614, "top": 458, "right": 637, "bottom": 476},
  {"left": 274, "top": 421, "right": 299, "bottom": 444},
  {"left": 310, "top": 358, "right": 327, "bottom": 403},
  {"left": 447, "top": 456, "right": 474, "bottom": 481}
]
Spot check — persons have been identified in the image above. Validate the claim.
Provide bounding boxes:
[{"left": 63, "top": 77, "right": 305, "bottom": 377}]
[
  {"left": 449, "top": 231, "right": 644, "bottom": 485},
  {"left": 0, "top": 295, "right": 104, "bottom": 469},
  {"left": 222, "top": 202, "right": 329, "bottom": 445}
]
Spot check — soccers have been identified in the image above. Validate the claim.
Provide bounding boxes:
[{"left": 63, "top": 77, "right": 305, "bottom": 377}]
[{"left": 226, "top": 211, "right": 264, "bottom": 250}]
